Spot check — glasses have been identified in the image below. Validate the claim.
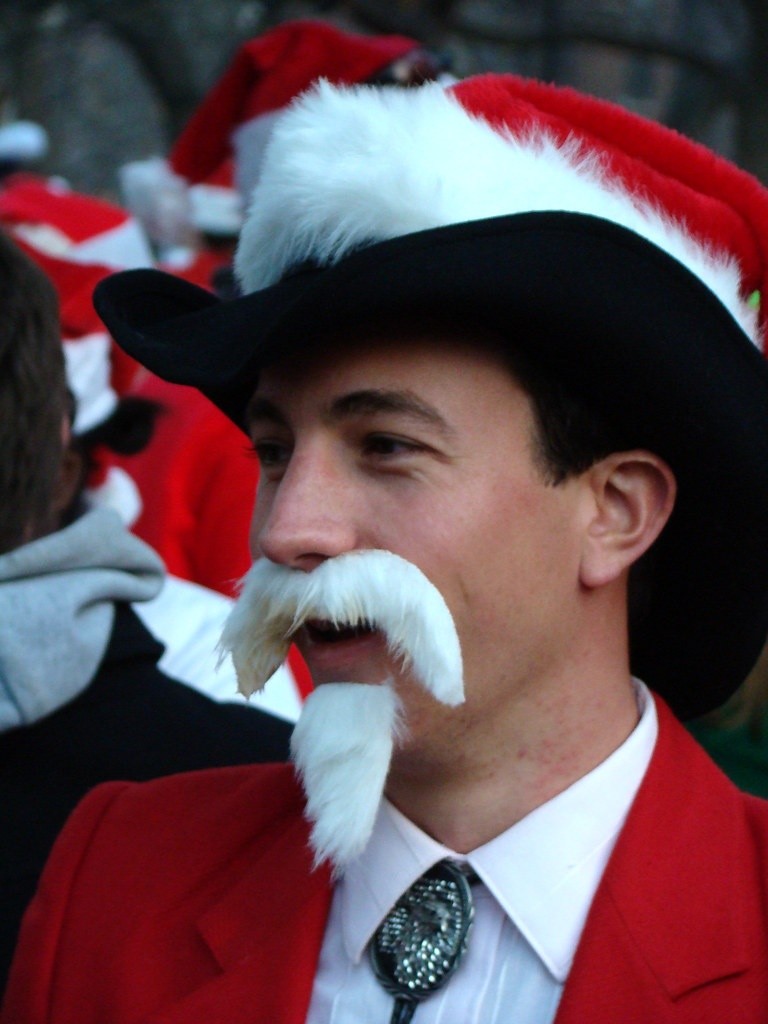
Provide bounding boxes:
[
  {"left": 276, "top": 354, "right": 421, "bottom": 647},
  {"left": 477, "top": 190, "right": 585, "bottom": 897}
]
[
  {"left": 64, "top": 385, "right": 77, "bottom": 427},
  {"left": 367, "top": 49, "right": 455, "bottom": 86}
]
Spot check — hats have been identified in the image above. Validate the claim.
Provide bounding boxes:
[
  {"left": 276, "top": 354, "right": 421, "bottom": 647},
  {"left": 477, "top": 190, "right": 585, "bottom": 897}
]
[
  {"left": 93, "top": 207, "right": 768, "bottom": 722},
  {"left": 0, "top": 172, "right": 153, "bottom": 277},
  {"left": 0, "top": 230, "right": 119, "bottom": 435},
  {"left": 121, "top": 20, "right": 459, "bottom": 234},
  {"left": 231, "top": 68, "right": 768, "bottom": 355}
]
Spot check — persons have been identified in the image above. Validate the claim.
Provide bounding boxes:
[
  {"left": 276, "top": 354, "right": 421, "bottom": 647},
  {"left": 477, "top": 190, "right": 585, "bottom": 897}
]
[
  {"left": 0, "top": 73, "right": 768, "bottom": 1024},
  {"left": 0, "top": 27, "right": 452, "bottom": 1008}
]
